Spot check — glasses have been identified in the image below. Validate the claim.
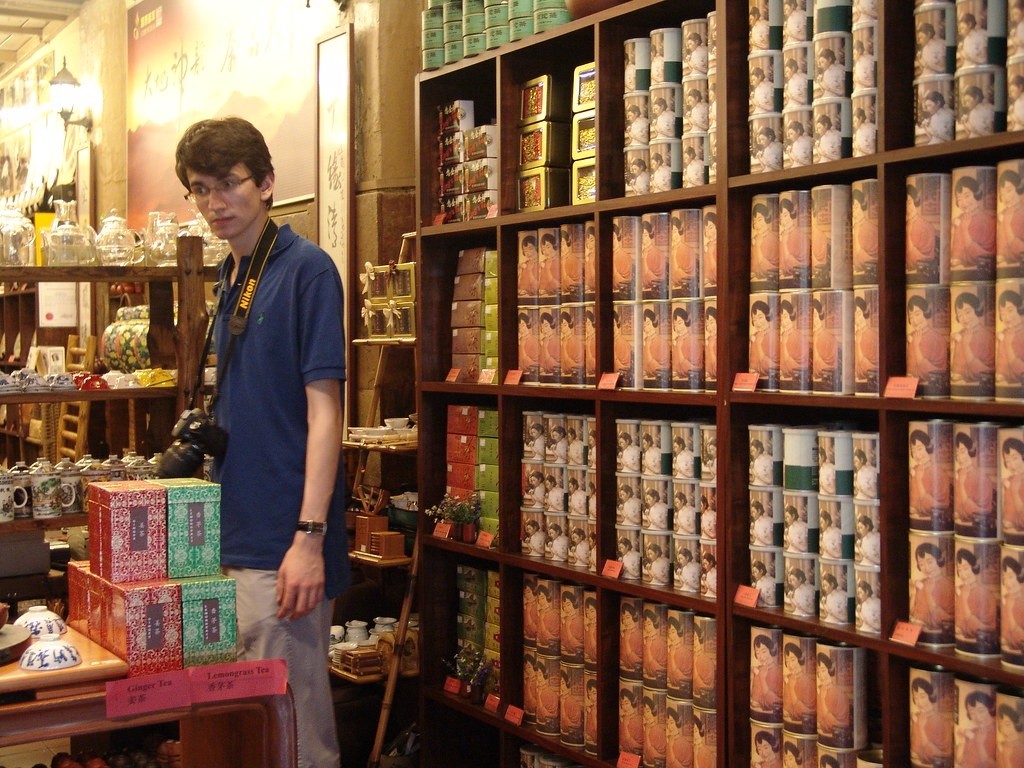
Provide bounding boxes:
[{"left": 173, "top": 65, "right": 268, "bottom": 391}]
[{"left": 184, "top": 173, "right": 259, "bottom": 205}]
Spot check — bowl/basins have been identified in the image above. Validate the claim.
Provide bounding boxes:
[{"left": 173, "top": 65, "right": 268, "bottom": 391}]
[
  {"left": 101, "top": 370, "right": 144, "bottom": 389},
  {"left": 0, "top": 603, "right": 10, "bottom": 630},
  {"left": 74, "top": 371, "right": 111, "bottom": 390},
  {"left": 133, "top": 368, "right": 176, "bottom": 387},
  {"left": 19, "top": 635, "right": 82, "bottom": 670},
  {"left": 44, "top": 375, "right": 76, "bottom": 391},
  {"left": 0, "top": 371, "right": 25, "bottom": 394},
  {"left": 13, "top": 606, "right": 68, "bottom": 638},
  {"left": 17, "top": 368, "right": 51, "bottom": 393}
]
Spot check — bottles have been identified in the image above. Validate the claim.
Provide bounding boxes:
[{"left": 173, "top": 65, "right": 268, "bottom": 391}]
[
  {"left": 145, "top": 212, "right": 190, "bottom": 266},
  {"left": 373, "top": 617, "right": 397, "bottom": 633},
  {"left": 88, "top": 208, "right": 146, "bottom": 266},
  {"left": 345, "top": 620, "right": 368, "bottom": 642},
  {"left": 369, "top": 629, "right": 378, "bottom": 645},
  {"left": 0, "top": 205, "right": 36, "bottom": 266},
  {"left": 41, "top": 200, "right": 95, "bottom": 266}
]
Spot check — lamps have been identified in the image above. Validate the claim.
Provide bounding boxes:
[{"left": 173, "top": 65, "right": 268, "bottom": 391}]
[{"left": 48, "top": 49, "right": 91, "bottom": 135}]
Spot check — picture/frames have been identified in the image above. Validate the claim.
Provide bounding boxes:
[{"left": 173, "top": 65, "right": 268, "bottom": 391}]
[{"left": 317, "top": 24, "right": 355, "bottom": 430}]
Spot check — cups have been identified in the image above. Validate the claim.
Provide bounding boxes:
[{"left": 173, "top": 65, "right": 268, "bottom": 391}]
[
  {"left": 348, "top": 427, "right": 392, "bottom": 442},
  {"left": 328, "top": 626, "right": 343, "bottom": 660},
  {"left": 409, "top": 413, "right": 418, "bottom": 424},
  {"left": 75, "top": 452, "right": 163, "bottom": 513},
  {"left": 0, "top": 457, "right": 81, "bottom": 523},
  {"left": 384, "top": 419, "right": 409, "bottom": 429}
]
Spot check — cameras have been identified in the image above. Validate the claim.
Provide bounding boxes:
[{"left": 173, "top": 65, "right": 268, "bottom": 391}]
[{"left": 157, "top": 408, "right": 229, "bottom": 480}]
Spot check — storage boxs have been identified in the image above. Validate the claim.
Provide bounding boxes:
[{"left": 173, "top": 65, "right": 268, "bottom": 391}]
[
  {"left": 148, "top": 478, "right": 219, "bottom": 576},
  {"left": 518, "top": 61, "right": 595, "bottom": 212},
  {"left": 68, "top": 560, "right": 182, "bottom": 670},
  {"left": 86, "top": 481, "right": 166, "bottom": 583},
  {"left": 366, "top": 262, "right": 415, "bottom": 337},
  {"left": 168, "top": 576, "right": 238, "bottom": 667}
]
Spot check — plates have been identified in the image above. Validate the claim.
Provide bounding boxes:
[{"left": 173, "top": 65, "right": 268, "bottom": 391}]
[
  {"left": 332, "top": 643, "right": 358, "bottom": 668},
  {"left": 0, "top": 624, "right": 31, "bottom": 651}
]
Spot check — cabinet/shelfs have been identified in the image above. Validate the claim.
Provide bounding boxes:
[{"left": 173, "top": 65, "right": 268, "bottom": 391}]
[
  {"left": 414, "top": 1, "right": 1024, "bottom": 767},
  {"left": 0, "top": 262, "right": 221, "bottom": 624},
  {"left": 327, "top": 333, "right": 417, "bottom": 766}
]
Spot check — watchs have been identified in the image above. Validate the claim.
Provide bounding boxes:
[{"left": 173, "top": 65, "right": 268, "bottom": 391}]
[{"left": 297, "top": 520, "right": 328, "bottom": 535}]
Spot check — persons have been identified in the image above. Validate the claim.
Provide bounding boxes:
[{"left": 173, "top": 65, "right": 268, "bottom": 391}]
[
  {"left": 514, "top": 411, "right": 883, "bottom": 634},
  {"left": 175, "top": 117, "right": 348, "bottom": 768},
  {"left": 618, "top": 0, "right": 1024, "bottom": 198}
]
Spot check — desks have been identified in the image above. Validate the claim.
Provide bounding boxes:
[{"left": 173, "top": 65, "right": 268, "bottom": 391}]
[{"left": 0, "top": 674, "right": 297, "bottom": 768}]
